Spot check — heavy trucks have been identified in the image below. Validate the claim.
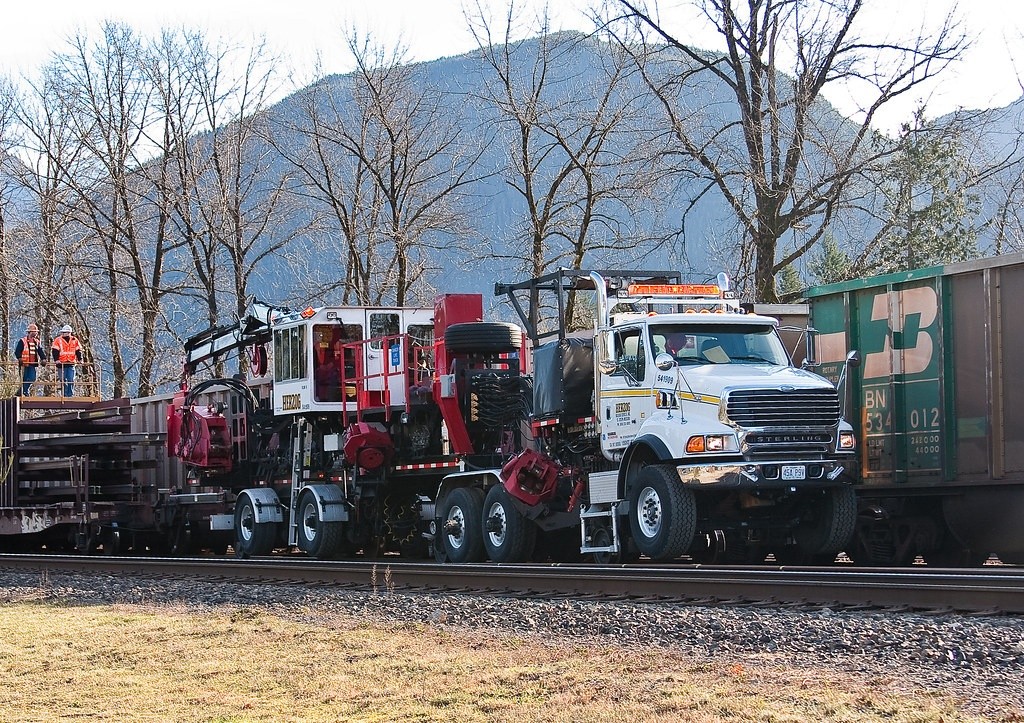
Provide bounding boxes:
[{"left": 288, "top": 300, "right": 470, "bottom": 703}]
[
  {"left": 157, "top": 294, "right": 531, "bottom": 556},
  {"left": 429, "top": 268, "right": 862, "bottom": 564}
]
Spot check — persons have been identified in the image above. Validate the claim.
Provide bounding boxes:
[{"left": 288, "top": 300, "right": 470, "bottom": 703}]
[
  {"left": 15, "top": 324, "right": 47, "bottom": 396},
  {"left": 51, "top": 325, "right": 84, "bottom": 397}
]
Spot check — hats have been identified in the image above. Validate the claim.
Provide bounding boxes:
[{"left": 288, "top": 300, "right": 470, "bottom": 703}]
[
  {"left": 26, "top": 324, "right": 39, "bottom": 332},
  {"left": 60, "top": 325, "right": 73, "bottom": 333}
]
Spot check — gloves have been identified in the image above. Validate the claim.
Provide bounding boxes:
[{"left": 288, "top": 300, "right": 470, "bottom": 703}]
[
  {"left": 40, "top": 360, "right": 46, "bottom": 366},
  {"left": 19, "top": 360, "right": 23, "bottom": 366}
]
